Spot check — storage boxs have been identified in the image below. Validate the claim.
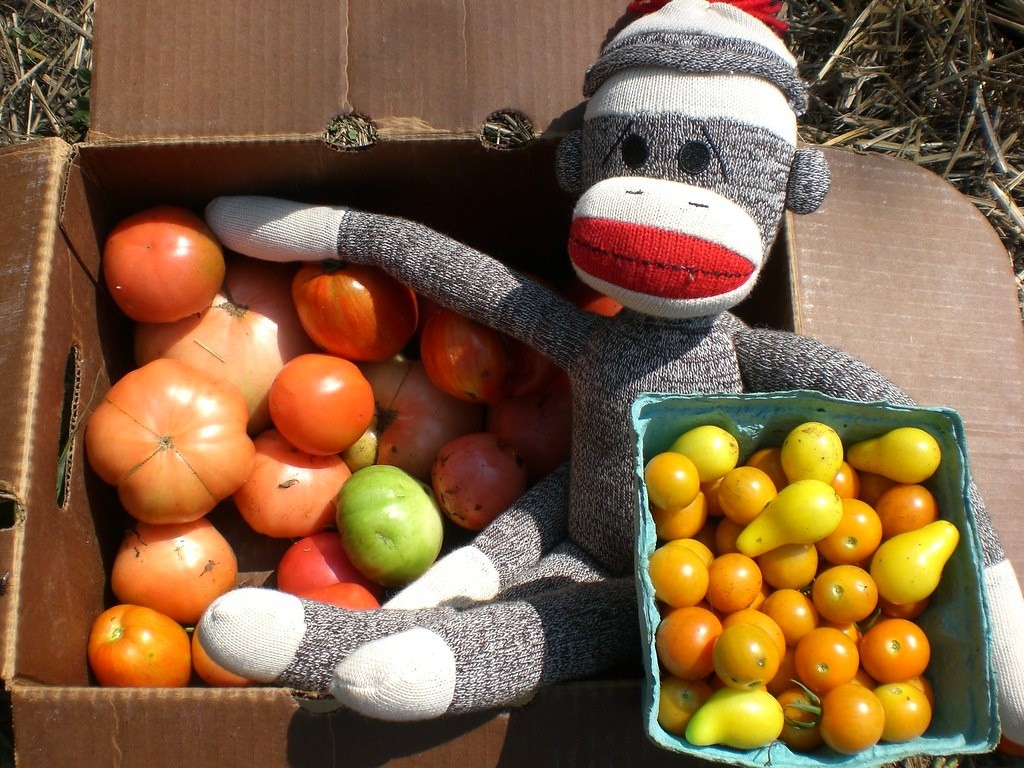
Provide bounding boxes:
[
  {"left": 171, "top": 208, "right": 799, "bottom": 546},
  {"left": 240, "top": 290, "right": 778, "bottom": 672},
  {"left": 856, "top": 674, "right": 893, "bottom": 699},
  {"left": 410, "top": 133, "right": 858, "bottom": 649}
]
[
  {"left": 629, "top": 388, "right": 1001, "bottom": 768},
  {"left": 0, "top": 0, "right": 1024, "bottom": 768}
]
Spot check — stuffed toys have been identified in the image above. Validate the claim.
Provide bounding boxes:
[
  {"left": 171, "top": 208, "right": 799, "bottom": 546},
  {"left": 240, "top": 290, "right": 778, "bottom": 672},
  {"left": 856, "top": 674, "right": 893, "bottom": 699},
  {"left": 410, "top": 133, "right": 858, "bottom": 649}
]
[{"left": 203, "top": 0, "right": 1024, "bottom": 747}]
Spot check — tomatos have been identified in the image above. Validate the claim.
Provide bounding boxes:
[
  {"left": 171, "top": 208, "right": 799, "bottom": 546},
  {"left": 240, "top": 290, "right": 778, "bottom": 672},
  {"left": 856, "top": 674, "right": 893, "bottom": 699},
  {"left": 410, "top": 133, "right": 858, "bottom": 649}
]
[
  {"left": 84, "top": 203, "right": 573, "bottom": 691},
  {"left": 644, "top": 421, "right": 960, "bottom": 757}
]
